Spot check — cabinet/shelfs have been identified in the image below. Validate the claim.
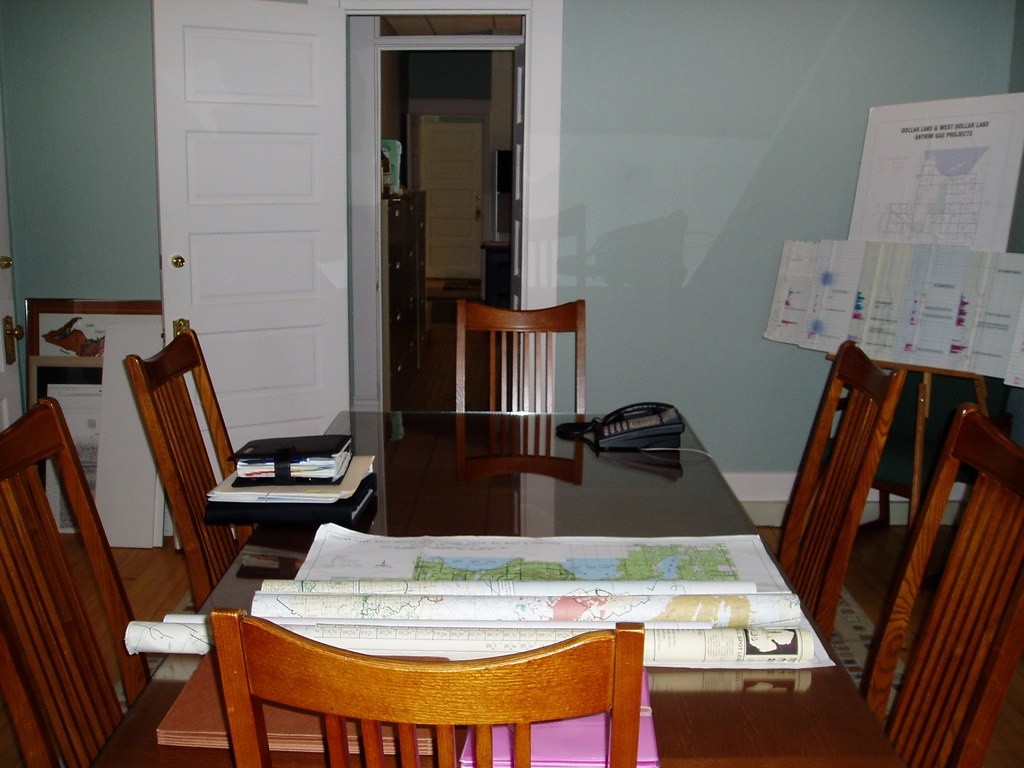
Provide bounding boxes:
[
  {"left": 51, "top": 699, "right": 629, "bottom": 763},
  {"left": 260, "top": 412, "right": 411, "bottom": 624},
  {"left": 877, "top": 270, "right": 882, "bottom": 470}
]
[
  {"left": 380, "top": 189, "right": 431, "bottom": 383},
  {"left": 480, "top": 240, "right": 512, "bottom": 308}
]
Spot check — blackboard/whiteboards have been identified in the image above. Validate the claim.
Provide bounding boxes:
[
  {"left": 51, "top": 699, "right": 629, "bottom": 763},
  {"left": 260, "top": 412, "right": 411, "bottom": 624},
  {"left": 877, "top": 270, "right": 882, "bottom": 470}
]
[{"left": 823, "top": 92, "right": 1024, "bottom": 381}]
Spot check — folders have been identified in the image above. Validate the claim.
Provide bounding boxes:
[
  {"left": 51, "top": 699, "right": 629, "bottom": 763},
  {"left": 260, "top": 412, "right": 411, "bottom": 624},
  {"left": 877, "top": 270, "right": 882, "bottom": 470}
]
[{"left": 203, "top": 473, "right": 378, "bottom": 526}]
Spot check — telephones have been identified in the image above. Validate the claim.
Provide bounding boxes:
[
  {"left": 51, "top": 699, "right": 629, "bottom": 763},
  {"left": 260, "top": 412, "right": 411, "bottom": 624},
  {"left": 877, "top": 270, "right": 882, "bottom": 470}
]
[{"left": 593, "top": 402, "right": 686, "bottom": 451}]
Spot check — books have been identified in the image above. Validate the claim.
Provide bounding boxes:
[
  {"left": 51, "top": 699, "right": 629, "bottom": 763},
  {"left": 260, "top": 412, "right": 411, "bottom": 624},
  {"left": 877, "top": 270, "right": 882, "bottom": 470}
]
[
  {"left": 202, "top": 436, "right": 379, "bottom": 528},
  {"left": 233, "top": 523, "right": 316, "bottom": 580},
  {"left": 155, "top": 647, "right": 454, "bottom": 757},
  {"left": 459, "top": 667, "right": 660, "bottom": 768}
]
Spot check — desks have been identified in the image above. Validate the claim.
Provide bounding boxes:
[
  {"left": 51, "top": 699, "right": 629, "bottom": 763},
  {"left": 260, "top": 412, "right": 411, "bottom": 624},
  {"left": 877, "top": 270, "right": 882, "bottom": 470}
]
[{"left": 90, "top": 411, "right": 901, "bottom": 768}]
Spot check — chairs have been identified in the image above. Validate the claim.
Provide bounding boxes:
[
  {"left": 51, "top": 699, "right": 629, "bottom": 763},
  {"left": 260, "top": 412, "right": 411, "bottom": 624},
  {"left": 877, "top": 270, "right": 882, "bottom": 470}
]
[
  {"left": 209, "top": 608, "right": 645, "bottom": 768},
  {"left": 124, "top": 327, "right": 256, "bottom": 610},
  {"left": 772, "top": 339, "right": 909, "bottom": 641},
  {"left": 0, "top": 397, "right": 150, "bottom": 768},
  {"left": 865, "top": 402, "right": 1024, "bottom": 768},
  {"left": 456, "top": 299, "right": 587, "bottom": 414}
]
